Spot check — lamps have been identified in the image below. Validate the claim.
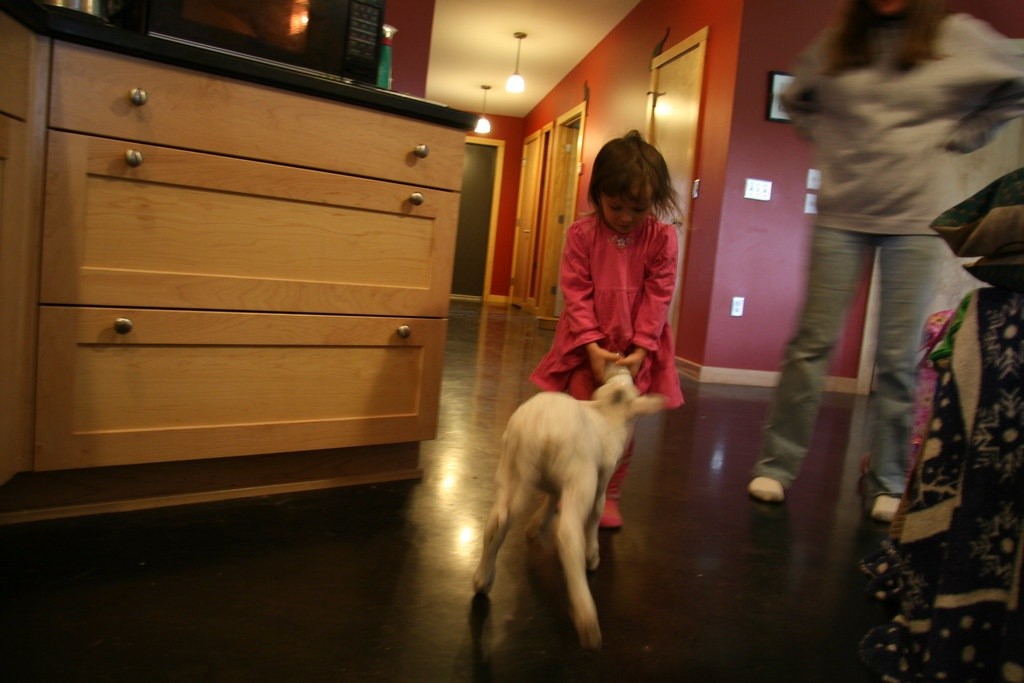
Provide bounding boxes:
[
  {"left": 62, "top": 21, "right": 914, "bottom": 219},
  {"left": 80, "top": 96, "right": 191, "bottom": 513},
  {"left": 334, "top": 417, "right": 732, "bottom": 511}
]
[
  {"left": 505, "top": 32, "right": 527, "bottom": 93},
  {"left": 474, "top": 86, "right": 492, "bottom": 134}
]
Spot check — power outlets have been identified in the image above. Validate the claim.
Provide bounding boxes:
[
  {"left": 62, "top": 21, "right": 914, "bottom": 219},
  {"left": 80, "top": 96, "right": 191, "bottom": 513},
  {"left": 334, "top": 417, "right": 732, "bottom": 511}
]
[
  {"left": 804, "top": 195, "right": 818, "bottom": 214},
  {"left": 731, "top": 296, "right": 744, "bottom": 316},
  {"left": 745, "top": 178, "right": 773, "bottom": 201},
  {"left": 807, "top": 169, "right": 820, "bottom": 190}
]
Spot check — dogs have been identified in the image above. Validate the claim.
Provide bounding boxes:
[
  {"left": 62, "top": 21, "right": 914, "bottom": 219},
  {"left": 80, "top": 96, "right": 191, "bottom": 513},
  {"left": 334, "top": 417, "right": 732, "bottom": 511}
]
[{"left": 472, "top": 353, "right": 672, "bottom": 657}]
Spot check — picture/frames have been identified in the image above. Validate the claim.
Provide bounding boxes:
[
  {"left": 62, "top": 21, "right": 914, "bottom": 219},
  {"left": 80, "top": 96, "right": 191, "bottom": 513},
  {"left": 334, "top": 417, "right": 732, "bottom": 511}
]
[{"left": 765, "top": 72, "right": 796, "bottom": 122}]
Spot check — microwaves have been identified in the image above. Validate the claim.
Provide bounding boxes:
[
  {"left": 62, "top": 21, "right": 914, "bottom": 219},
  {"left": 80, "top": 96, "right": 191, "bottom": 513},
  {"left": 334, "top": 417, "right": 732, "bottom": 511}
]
[{"left": 134, "top": 0, "right": 386, "bottom": 88}]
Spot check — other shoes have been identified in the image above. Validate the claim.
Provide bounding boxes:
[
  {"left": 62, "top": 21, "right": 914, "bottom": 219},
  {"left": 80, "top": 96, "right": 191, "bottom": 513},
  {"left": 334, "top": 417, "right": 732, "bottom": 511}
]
[{"left": 598, "top": 497, "right": 624, "bottom": 529}]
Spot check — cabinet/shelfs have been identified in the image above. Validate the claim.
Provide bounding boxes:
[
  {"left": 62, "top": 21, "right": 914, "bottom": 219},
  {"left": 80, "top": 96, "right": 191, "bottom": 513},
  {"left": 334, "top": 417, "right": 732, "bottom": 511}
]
[{"left": 0, "top": 0, "right": 479, "bottom": 523}]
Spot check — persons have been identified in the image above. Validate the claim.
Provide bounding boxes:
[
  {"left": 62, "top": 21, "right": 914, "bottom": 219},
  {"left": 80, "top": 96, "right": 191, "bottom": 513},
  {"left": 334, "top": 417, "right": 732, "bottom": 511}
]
[
  {"left": 741, "top": 0, "right": 1024, "bottom": 522},
  {"left": 531, "top": 130, "right": 686, "bottom": 527}
]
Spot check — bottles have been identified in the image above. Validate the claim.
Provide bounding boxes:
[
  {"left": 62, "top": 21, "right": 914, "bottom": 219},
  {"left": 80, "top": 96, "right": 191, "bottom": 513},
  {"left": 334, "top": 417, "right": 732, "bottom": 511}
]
[{"left": 376, "top": 22, "right": 397, "bottom": 91}]
